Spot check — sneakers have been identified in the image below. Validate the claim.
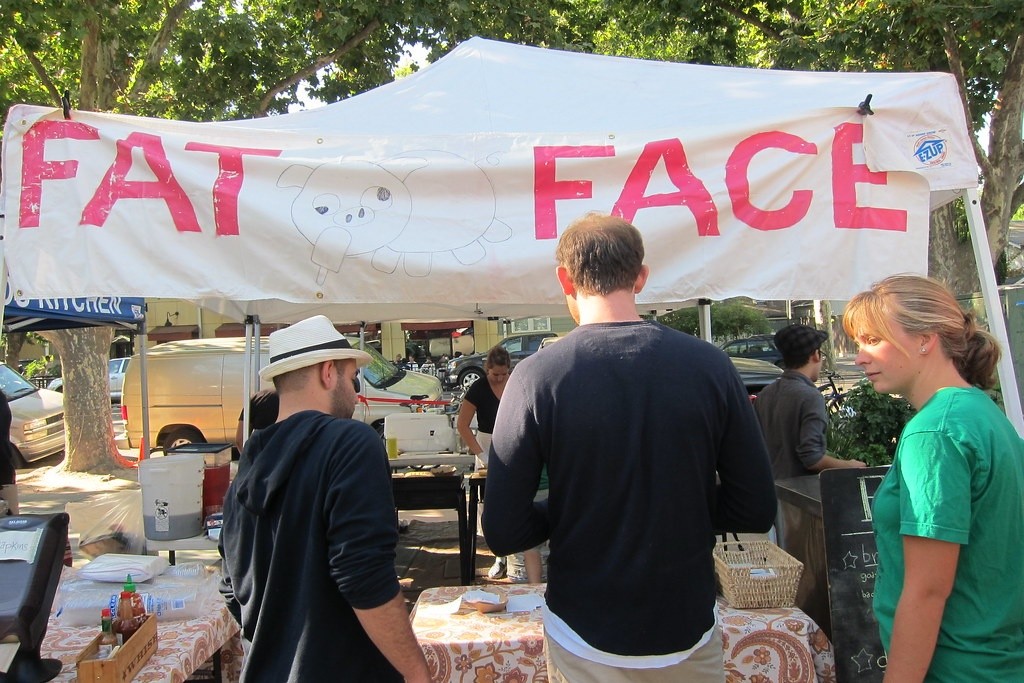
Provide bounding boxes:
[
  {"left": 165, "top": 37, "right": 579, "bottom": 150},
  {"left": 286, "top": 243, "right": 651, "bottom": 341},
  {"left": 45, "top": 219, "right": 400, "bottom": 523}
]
[{"left": 488, "top": 557, "right": 507, "bottom": 580}]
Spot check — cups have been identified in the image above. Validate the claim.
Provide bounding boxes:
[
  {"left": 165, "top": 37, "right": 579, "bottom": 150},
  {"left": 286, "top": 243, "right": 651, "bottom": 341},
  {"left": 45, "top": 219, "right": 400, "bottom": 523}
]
[
  {"left": 167, "top": 565, "right": 201, "bottom": 579},
  {"left": 385, "top": 437, "right": 397, "bottom": 460},
  {"left": 61, "top": 588, "right": 197, "bottom": 617}
]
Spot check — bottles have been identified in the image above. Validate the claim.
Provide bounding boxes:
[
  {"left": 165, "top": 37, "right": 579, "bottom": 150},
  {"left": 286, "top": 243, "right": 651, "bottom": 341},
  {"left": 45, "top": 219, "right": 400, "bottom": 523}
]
[{"left": 101, "top": 574, "right": 147, "bottom": 654}]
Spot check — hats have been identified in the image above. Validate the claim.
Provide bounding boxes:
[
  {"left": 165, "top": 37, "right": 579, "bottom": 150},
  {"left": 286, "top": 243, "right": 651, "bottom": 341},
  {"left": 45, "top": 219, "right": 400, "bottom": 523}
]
[
  {"left": 774, "top": 323, "right": 829, "bottom": 358},
  {"left": 257, "top": 315, "right": 375, "bottom": 382}
]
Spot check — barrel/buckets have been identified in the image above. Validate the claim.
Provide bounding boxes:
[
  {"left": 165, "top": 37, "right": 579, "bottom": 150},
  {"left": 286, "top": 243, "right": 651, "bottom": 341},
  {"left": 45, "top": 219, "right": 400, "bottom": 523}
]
[{"left": 138, "top": 454, "right": 205, "bottom": 540}]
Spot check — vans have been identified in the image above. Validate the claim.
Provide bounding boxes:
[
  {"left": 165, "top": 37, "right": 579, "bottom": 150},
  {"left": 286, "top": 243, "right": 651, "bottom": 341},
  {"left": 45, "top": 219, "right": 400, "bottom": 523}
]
[{"left": 113, "top": 337, "right": 447, "bottom": 469}]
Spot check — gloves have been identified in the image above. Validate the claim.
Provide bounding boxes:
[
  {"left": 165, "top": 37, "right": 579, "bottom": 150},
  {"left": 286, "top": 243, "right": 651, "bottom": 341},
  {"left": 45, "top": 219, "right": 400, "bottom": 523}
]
[{"left": 478, "top": 452, "right": 489, "bottom": 469}]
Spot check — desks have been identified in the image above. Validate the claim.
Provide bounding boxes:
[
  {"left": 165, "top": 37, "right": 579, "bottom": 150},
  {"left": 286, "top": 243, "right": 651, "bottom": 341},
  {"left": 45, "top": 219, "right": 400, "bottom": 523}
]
[
  {"left": 467, "top": 472, "right": 488, "bottom": 584},
  {"left": 391, "top": 468, "right": 467, "bottom": 586},
  {"left": 146, "top": 530, "right": 220, "bottom": 566},
  {"left": 403, "top": 582, "right": 838, "bottom": 683},
  {"left": 0, "top": 569, "right": 244, "bottom": 683}
]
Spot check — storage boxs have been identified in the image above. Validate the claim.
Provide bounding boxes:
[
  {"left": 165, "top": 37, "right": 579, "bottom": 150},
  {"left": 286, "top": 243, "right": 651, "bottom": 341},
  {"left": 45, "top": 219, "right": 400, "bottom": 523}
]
[
  {"left": 76, "top": 611, "right": 158, "bottom": 683},
  {"left": 384, "top": 413, "right": 450, "bottom": 452},
  {"left": 165, "top": 441, "right": 233, "bottom": 467}
]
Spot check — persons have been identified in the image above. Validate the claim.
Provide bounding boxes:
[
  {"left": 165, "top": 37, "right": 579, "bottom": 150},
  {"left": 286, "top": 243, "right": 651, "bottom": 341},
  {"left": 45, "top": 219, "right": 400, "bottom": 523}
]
[
  {"left": 842, "top": 276, "right": 1024, "bottom": 683},
  {"left": 0, "top": 391, "right": 20, "bottom": 514},
  {"left": 506, "top": 464, "right": 550, "bottom": 583},
  {"left": 392, "top": 352, "right": 461, "bottom": 370},
  {"left": 457, "top": 346, "right": 510, "bottom": 579},
  {"left": 753, "top": 324, "right": 867, "bottom": 478},
  {"left": 480, "top": 215, "right": 777, "bottom": 683},
  {"left": 217, "top": 314, "right": 431, "bottom": 683},
  {"left": 236, "top": 387, "right": 279, "bottom": 455}
]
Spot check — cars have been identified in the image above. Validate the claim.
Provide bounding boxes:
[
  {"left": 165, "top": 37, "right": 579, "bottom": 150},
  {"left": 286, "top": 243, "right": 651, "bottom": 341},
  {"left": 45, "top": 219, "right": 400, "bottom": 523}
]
[
  {"left": 0, "top": 361, "right": 67, "bottom": 469},
  {"left": 47, "top": 356, "right": 131, "bottom": 403}
]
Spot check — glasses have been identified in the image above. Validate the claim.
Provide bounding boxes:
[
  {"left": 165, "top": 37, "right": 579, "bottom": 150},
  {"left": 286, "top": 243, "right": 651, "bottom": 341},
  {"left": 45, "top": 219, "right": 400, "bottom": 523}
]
[{"left": 819, "top": 350, "right": 827, "bottom": 361}]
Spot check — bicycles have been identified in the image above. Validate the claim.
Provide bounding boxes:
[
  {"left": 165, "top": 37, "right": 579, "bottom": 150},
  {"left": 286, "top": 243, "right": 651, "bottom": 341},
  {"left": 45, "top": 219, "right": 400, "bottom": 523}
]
[
  {"left": 815, "top": 370, "right": 851, "bottom": 418},
  {"left": 445, "top": 379, "right": 474, "bottom": 416}
]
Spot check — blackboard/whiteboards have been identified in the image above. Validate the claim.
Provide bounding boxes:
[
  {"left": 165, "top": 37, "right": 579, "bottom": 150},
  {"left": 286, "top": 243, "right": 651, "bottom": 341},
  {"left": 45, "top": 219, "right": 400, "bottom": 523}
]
[{"left": 819, "top": 467, "right": 893, "bottom": 683}]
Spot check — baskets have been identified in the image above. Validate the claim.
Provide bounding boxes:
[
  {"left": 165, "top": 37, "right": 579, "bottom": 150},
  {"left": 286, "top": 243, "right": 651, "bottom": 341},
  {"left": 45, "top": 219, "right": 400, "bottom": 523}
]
[{"left": 713, "top": 540, "right": 804, "bottom": 610}]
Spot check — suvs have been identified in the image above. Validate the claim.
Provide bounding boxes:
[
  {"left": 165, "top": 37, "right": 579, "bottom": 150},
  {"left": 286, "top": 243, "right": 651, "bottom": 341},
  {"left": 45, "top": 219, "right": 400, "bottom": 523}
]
[
  {"left": 718, "top": 333, "right": 788, "bottom": 402},
  {"left": 444, "top": 331, "right": 560, "bottom": 394}
]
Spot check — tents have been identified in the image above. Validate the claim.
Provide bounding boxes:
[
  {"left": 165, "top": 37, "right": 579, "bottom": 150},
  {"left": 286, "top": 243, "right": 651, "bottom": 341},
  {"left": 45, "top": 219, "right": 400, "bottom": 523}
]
[
  {"left": 3, "top": 274, "right": 150, "bottom": 458},
  {"left": 0, "top": 35, "right": 1024, "bottom": 466}
]
